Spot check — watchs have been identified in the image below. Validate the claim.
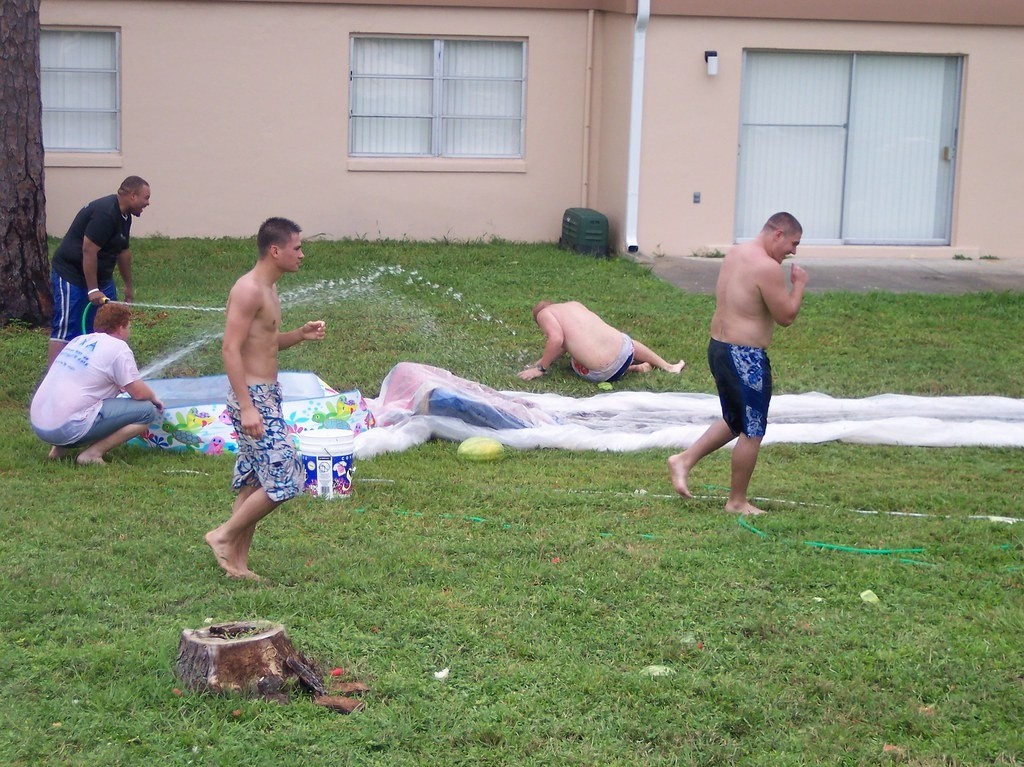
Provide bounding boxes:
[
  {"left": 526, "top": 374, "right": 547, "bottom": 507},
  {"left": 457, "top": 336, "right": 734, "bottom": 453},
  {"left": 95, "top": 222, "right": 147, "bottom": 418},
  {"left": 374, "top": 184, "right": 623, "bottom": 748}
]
[{"left": 537, "top": 363, "right": 547, "bottom": 372}]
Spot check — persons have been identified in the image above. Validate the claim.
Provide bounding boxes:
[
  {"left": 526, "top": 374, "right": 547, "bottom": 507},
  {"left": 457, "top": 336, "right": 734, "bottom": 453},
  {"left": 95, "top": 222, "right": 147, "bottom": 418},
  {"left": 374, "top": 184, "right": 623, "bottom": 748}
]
[
  {"left": 517, "top": 299, "right": 686, "bottom": 386},
  {"left": 203, "top": 217, "right": 326, "bottom": 581},
  {"left": 30, "top": 303, "right": 165, "bottom": 465},
  {"left": 48, "top": 175, "right": 151, "bottom": 373},
  {"left": 667, "top": 212, "right": 809, "bottom": 515}
]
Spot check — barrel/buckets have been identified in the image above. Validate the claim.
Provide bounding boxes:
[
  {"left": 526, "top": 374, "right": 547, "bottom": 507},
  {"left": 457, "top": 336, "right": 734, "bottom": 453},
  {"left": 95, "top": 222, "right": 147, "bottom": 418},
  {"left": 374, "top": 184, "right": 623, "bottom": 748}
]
[{"left": 295, "top": 429, "right": 354, "bottom": 497}]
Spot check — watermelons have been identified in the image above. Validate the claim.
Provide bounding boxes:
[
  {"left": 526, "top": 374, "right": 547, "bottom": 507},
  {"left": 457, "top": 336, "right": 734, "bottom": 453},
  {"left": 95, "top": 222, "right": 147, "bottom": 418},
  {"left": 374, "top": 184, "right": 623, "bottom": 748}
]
[{"left": 456, "top": 435, "right": 503, "bottom": 461}]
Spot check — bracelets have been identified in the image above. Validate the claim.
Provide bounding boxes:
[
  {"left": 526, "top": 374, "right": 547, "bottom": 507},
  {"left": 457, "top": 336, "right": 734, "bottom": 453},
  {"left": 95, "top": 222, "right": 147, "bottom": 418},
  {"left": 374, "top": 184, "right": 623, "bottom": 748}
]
[{"left": 88, "top": 288, "right": 99, "bottom": 296}]
